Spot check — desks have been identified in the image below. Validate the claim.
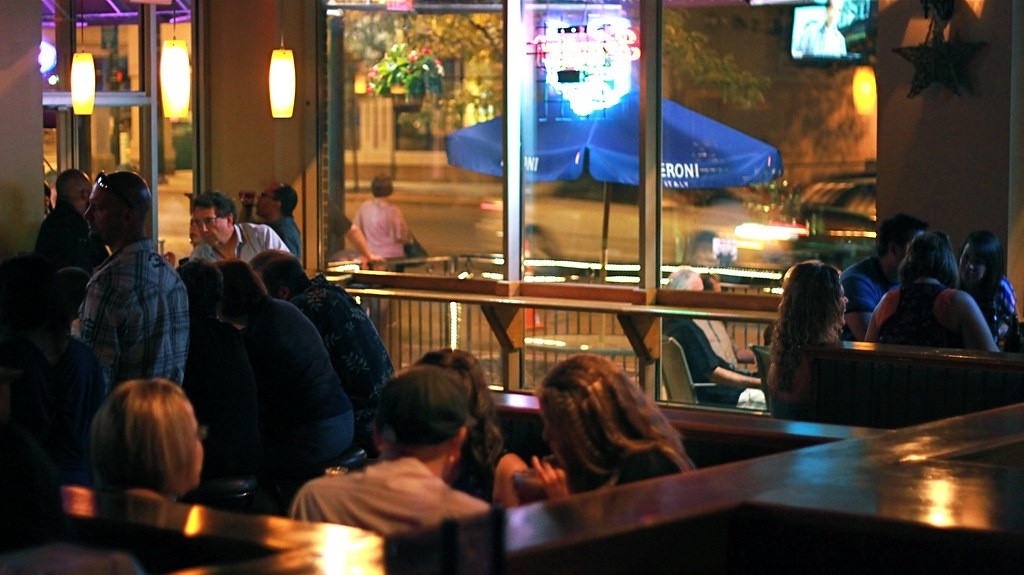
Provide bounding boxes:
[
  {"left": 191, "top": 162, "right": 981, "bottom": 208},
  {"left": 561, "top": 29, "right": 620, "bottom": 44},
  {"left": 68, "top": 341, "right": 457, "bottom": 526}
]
[{"left": 523, "top": 334, "right": 639, "bottom": 392}]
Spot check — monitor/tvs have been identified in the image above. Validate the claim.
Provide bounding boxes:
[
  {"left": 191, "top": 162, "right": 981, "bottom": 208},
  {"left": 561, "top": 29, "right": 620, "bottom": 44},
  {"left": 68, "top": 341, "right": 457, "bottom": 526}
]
[{"left": 789, "top": 2, "right": 867, "bottom": 65}]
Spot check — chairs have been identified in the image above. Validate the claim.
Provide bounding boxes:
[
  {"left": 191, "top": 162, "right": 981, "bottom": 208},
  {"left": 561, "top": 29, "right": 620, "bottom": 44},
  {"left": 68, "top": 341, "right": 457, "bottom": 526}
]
[
  {"left": 662, "top": 335, "right": 718, "bottom": 406},
  {"left": 751, "top": 345, "right": 808, "bottom": 421}
]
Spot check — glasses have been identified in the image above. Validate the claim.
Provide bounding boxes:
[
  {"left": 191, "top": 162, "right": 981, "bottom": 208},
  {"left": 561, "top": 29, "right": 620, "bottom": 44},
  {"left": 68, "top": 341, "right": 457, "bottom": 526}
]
[
  {"left": 97, "top": 170, "right": 110, "bottom": 191},
  {"left": 190, "top": 212, "right": 221, "bottom": 228},
  {"left": 199, "top": 427, "right": 208, "bottom": 438},
  {"left": 260, "top": 192, "right": 270, "bottom": 198}
]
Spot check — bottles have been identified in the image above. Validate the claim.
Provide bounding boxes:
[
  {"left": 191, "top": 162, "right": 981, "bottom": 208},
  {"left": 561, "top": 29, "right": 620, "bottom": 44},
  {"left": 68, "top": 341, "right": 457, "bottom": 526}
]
[{"left": 740, "top": 167, "right": 803, "bottom": 227}]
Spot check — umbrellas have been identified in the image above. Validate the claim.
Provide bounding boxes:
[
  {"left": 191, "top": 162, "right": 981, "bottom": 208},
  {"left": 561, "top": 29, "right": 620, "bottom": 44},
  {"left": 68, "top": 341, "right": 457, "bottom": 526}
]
[{"left": 444, "top": 88, "right": 784, "bottom": 341}]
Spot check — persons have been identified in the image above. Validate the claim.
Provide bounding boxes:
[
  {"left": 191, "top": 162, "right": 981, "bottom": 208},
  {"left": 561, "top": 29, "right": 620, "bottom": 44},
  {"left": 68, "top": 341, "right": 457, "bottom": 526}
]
[
  {"left": 662, "top": 265, "right": 762, "bottom": 406},
  {"left": 250, "top": 249, "right": 397, "bottom": 429},
  {"left": 91, "top": 378, "right": 204, "bottom": 502},
  {"left": 35, "top": 170, "right": 108, "bottom": 269},
  {"left": 176, "top": 260, "right": 265, "bottom": 480},
  {"left": 864, "top": 230, "right": 1000, "bottom": 352},
  {"left": 957, "top": 230, "right": 1016, "bottom": 340},
  {"left": 288, "top": 364, "right": 490, "bottom": 537},
  {"left": 768, "top": 260, "right": 849, "bottom": 393},
  {"left": 238, "top": 181, "right": 302, "bottom": 260},
  {"left": 839, "top": 213, "right": 929, "bottom": 342},
  {"left": 803, "top": 0, "right": 847, "bottom": 56},
  {"left": 510, "top": 354, "right": 694, "bottom": 506},
  {"left": 416, "top": 349, "right": 528, "bottom": 509},
  {"left": 213, "top": 258, "right": 354, "bottom": 485},
  {"left": 0, "top": 254, "right": 106, "bottom": 470},
  {"left": 78, "top": 171, "right": 190, "bottom": 397},
  {"left": 164, "top": 192, "right": 290, "bottom": 266},
  {"left": 351, "top": 173, "right": 409, "bottom": 270}
]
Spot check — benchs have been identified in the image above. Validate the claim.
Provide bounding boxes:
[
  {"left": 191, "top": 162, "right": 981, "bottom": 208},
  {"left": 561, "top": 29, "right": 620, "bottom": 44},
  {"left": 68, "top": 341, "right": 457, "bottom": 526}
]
[{"left": 58, "top": 340, "right": 1024, "bottom": 575}]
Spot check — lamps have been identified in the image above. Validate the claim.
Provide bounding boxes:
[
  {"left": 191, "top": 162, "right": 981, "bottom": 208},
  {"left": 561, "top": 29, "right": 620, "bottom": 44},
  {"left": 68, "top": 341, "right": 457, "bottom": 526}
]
[
  {"left": 269, "top": 1, "right": 297, "bottom": 117},
  {"left": 70, "top": 0, "right": 96, "bottom": 115},
  {"left": 158, "top": 2, "right": 190, "bottom": 117}
]
